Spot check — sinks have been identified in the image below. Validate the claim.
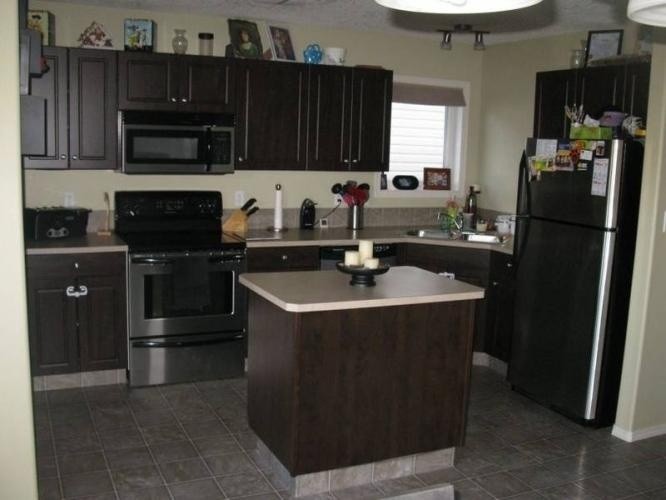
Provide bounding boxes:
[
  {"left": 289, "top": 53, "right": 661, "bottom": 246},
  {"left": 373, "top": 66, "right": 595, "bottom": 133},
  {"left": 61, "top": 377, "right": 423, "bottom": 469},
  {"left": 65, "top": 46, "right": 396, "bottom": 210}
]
[{"left": 407, "top": 228, "right": 476, "bottom": 241}]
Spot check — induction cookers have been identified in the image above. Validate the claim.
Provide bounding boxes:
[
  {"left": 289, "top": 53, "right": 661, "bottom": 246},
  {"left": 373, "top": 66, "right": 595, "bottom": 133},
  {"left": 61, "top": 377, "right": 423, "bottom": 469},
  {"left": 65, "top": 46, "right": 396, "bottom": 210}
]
[{"left": 120, "top": 228, "right": 245, "bottom": 249}]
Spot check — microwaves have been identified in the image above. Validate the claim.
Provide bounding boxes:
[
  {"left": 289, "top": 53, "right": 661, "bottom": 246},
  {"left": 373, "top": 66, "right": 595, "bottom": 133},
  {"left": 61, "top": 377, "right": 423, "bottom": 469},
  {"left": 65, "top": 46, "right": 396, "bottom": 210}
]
[{"left": 123, "top": 123, "right": 235, "bottom": 172}]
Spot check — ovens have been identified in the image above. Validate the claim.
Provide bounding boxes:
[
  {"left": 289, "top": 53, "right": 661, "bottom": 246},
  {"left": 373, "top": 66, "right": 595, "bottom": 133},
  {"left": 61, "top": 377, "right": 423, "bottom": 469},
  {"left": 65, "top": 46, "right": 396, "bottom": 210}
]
[{"left": 130, "top": 256, "right": 243, "bottom": 337}]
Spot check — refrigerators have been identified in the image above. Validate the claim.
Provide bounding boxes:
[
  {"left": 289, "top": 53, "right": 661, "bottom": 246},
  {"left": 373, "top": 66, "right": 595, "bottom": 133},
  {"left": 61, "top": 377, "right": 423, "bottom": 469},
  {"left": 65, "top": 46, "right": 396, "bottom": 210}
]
[{"left": 506, "top": 135, "right": 628, "bottom": 422}]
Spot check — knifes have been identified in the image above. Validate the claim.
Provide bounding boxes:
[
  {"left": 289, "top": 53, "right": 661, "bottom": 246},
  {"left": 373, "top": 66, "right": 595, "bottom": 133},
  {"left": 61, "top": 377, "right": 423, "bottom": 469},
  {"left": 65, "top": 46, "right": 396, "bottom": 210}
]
[{"left": 222, "top": 198, "right": 259, "bottom": 232}]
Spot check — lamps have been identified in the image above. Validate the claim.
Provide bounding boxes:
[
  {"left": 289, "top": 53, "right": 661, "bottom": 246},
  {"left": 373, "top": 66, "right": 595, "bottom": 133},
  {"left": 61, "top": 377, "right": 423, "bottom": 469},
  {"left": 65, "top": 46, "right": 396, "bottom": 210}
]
[{"left": 436, "top": 23, "right": 491, "bottom": 50}]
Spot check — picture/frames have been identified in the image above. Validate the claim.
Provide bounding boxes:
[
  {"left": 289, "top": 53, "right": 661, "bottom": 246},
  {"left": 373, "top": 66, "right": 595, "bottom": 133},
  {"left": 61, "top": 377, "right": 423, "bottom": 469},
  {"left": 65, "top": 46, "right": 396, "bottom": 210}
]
[
  {"left": 228, "top": 17, "right": 296, "bottom": 60},
  {"left": 423, "top": 167, "right": 450, "bottom": 190}
]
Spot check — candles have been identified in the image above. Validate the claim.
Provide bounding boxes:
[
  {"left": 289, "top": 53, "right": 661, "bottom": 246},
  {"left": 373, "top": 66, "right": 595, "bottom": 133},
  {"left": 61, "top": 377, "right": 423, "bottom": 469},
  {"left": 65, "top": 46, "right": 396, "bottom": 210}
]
[
  {"left": 273, "top": 190, "right": 283, "bottom": 230},
  {"left": 343, "top": 239, "right": 380, "bottom": 269}
]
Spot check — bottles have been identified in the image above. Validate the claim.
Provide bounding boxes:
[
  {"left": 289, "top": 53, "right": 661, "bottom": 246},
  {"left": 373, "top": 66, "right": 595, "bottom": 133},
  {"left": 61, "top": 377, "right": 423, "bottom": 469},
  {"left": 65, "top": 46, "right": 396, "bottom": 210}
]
[
  {"left": 466, "top": 186, "right": 477, "bottom": 224},
  {"left": 198, "top": 33, "right": 214, "bottom": 57},
  {"left": 172, "top": 29, "right": 188, "bottom": 54}
]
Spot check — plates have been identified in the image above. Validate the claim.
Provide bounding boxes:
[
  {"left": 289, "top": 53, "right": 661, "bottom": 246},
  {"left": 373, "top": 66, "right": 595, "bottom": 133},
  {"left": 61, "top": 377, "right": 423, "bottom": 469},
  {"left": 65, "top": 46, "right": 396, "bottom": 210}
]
[{"left": 336, "top": 259, "right": 391, "bottom": 276}]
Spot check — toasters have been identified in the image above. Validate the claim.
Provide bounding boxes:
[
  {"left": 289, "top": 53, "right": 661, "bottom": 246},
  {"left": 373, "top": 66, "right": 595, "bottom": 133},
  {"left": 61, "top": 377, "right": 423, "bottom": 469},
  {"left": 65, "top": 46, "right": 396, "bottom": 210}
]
[{"left": 26, "top": 205, "right": 92, "bottom": 240}]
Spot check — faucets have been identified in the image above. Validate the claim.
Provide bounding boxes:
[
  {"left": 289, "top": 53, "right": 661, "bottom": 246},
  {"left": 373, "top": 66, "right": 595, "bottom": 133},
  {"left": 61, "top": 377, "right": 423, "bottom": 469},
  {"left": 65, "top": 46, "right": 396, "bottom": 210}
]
[{"left": 436, "top": 212, "right": 463, "bottom": 231}]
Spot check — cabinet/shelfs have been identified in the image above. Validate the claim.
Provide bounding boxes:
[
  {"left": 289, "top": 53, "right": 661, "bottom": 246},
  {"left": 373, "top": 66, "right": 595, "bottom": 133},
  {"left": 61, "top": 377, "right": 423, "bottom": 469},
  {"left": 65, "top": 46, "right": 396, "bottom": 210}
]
[
  {"left": 119, "top": 50, "right": 238, "bottom": 115},
  {"left": 532, "top": 61, "right": 649, "bottom": 140},
  {"left": 22, "top": 44, "right": 119, "bottom": 170},
  {"left": 235, "top": 59, "right": 307, "bottom": 169},
  {"left": 308, "top": 63, "right": 393, "bottom": 172},
  {"left": 25, "top": 251, "right": 130, "bottom": 377},
  {"left": 244, "top": 246, "right": 506, "bottom": 286}
]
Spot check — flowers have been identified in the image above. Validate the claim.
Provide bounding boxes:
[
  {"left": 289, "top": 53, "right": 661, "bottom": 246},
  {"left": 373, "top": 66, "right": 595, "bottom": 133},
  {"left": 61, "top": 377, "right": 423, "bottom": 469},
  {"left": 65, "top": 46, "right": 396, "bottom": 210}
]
[{"left": 340, "top": 180, "right": 369, "bottom": 207}]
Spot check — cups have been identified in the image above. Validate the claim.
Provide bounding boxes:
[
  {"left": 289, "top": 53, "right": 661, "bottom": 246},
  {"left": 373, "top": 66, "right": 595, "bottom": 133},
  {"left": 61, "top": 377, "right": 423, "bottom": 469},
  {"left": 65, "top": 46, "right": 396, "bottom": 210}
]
[
  {"left": 463, "top": 213, "right": 472, "bottom": 227},
  {"left": 63, "top": 193, "right": 75, "bottom": 208}
]
